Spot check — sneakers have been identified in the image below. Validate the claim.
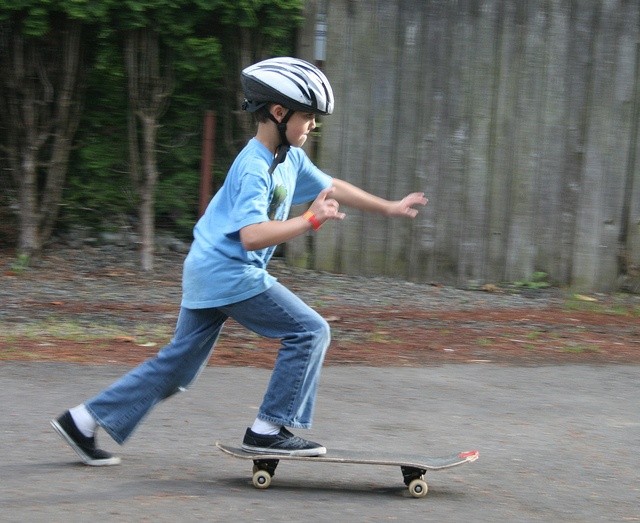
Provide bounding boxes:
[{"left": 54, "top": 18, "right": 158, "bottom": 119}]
[
  {"left": 49, "top": 409, "right": 120, "bottom": 466},
  {"left": 242, "top": 425, "right": 327, "bottom": 457}
]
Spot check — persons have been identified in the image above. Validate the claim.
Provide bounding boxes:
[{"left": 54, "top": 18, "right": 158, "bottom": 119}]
[{"left": 49, "top": 57, "right": 430, "bottom": 467}]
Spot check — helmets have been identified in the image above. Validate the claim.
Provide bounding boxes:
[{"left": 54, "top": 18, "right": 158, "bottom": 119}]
[{"left": 241, "top": 56, "right": 335, "bottom": 116}]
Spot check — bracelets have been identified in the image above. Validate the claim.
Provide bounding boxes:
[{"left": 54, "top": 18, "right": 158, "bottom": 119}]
[{"left": 302, "top": 210, "right": 321, "bottom": 230}]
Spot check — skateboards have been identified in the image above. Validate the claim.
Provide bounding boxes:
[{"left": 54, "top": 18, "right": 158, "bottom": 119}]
[{"left": 215, "top": 441, "right": 480, "bottom": 497}]
